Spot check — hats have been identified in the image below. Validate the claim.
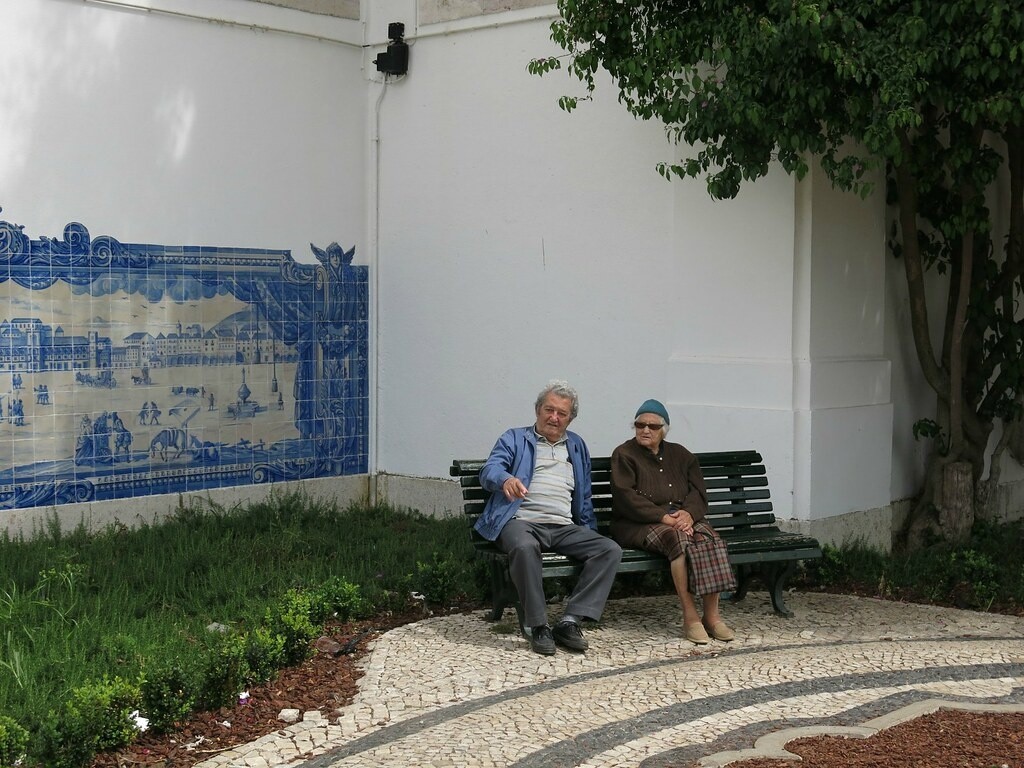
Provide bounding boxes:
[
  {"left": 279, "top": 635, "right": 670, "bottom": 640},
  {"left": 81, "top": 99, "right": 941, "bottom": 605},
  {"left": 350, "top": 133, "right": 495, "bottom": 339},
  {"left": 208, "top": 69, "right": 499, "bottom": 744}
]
[{"left": 635, "top": 399, "right": 669, "bottom": 425}]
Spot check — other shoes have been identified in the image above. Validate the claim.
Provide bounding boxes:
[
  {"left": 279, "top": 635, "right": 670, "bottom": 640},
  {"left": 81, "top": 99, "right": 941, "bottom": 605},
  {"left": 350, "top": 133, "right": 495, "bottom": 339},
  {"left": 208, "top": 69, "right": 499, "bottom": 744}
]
[
  {"left": 684, "top": 622, "right": 710, "bottom": 643},
  {"left": 702, "top": 620, "right": 735, "bottom": 641}
]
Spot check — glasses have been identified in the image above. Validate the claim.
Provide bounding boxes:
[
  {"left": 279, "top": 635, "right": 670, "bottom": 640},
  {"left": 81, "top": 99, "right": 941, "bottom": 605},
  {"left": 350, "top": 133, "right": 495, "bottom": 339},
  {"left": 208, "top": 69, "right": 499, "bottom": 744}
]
[{"left": 634, "top": 421, "right": 664, "bottom": 430}]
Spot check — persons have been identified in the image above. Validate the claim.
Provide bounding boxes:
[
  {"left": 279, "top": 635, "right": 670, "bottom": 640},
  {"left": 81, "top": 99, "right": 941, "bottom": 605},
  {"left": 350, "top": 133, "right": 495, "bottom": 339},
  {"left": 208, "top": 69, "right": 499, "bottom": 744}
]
[
  {"left": 609, "top": 399, "right": 736, "bottom": 643},
  {"left": 473, "top": 384, "right": 623, "bottom": 654}
]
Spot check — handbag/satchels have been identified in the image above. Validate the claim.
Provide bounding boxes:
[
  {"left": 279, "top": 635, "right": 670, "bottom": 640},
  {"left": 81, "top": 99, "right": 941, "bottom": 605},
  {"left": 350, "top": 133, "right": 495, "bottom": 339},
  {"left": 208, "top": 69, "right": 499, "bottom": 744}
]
[{"left": 686, "top": 531, "right": 737, "bottom": 596}]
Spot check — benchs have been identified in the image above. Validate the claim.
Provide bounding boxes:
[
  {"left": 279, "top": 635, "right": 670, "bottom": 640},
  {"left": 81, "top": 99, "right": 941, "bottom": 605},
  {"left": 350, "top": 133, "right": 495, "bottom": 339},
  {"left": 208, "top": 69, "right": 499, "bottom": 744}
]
[{"left": 449, "top": 449, "right": 827, "bottom": 637}]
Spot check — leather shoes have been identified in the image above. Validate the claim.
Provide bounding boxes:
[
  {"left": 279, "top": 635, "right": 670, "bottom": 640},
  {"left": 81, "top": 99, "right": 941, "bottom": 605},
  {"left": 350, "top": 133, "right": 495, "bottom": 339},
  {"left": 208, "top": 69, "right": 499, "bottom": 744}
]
[
  {"left": 552, "top": 621, "right": 589, "bottom": 650},
  {"left": 531, "top": 625, "right": 556, "bottom": 654}
]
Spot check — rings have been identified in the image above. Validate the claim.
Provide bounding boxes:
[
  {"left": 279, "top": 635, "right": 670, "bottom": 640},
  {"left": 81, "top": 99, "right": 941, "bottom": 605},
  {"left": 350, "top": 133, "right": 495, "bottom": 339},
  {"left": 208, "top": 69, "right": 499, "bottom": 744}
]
[{"left": 684, "top": 522, "right": 688, "bottom": 527}]
